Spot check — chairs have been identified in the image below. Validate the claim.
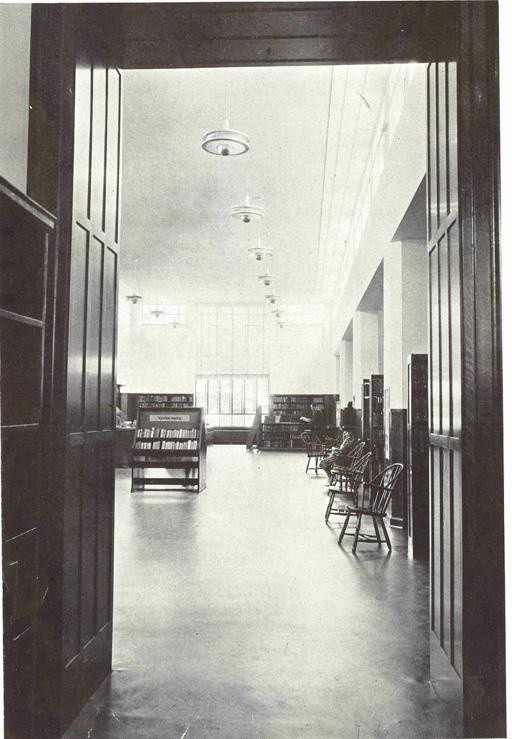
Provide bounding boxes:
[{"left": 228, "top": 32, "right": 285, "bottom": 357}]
[{"left": 298, "top": 423, "right": 406, "bottom": 557}]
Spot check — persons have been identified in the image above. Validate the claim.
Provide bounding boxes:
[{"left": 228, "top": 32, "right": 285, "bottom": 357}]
[{"left": 319, "top": 426, "right": 356, "bottom": 488}]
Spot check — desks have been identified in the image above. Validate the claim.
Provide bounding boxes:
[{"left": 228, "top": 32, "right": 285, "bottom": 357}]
[{"left": 116, "top": 423, "right": 140, "bottom": 471}]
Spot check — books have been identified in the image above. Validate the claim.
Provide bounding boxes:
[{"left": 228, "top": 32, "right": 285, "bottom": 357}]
[
  {"left": 267, "top": 396, "right": 324, "bottom": 449},
  {"left": 134, "top": 395, "right": 198, "bottom": 452}
]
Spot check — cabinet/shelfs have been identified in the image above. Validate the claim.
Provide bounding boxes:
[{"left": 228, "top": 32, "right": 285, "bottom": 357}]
[
  {"left": 129, "top": 406, "right": 208, "bottom": 493},
  {"left": 268, "top": 393, "right": 339, "bottom": 429},
  {"left": 133, "top": 392, "right": 194, "bottom": 410},
  {"left": 258, "top": 419, "right": 312, "bottom": 452},
  {"left": 400, "top": 352, "right": 438, "bottom": 564},
  {"left": 360, "top": 373, "right": 386, "bottom": 501},
  {"left": 386, "top": 407, "right": 406, "bottom": 530}
]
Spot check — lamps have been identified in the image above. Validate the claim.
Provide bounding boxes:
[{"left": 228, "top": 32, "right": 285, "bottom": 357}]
[
  {"left": 200, "top": 113, "right": 289, "bottom": 333},
  {"left": 124, "top": 287, "right": 180, "bottom": 330}
]
